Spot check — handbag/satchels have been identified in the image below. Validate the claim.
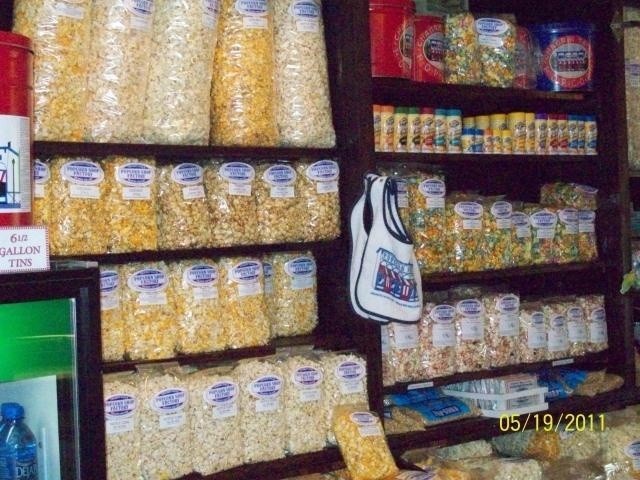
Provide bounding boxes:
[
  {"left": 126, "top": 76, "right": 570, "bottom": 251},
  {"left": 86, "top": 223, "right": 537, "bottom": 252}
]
[{"left": 348, "top": 172, "right": 424, "bottom": 324}]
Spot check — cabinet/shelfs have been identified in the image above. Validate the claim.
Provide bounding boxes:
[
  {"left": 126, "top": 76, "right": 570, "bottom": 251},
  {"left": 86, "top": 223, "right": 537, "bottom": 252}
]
[
  {"left": 614, "top": 0, "right": 639, "bottom": 402},
  {"left": 354, "top": 2, "right": 636, "bottom": 460},
  {"left": 1, "top": 0, "right": 357, "bottom": 480}
]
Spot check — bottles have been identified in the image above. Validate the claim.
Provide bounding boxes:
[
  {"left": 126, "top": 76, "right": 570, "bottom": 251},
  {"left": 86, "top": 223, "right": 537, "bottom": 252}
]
[
  {"left": 373, "top": 102, "right": 597, "bottom": 154},
  {"left": 0, "top": 403, "right": 44, "bottom": 479}
]
